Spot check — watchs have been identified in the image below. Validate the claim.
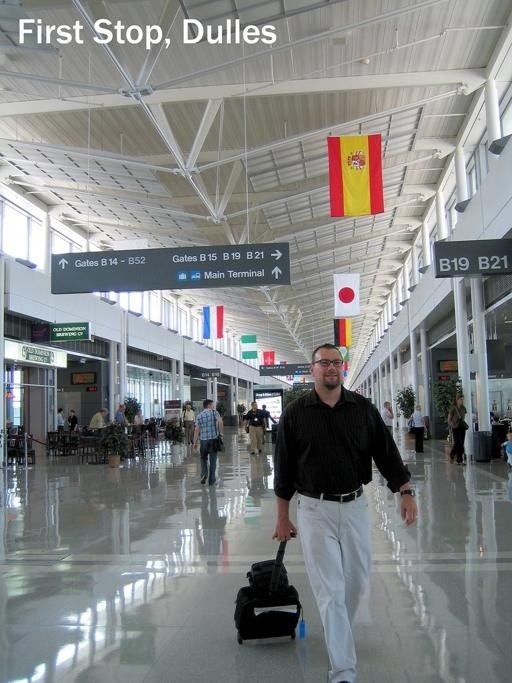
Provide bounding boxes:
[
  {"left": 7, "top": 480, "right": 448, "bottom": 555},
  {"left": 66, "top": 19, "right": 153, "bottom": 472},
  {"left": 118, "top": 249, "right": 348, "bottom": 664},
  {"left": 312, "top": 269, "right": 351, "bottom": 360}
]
[{"left": 399, "top": 487, "right": 420, "bottom": 499}]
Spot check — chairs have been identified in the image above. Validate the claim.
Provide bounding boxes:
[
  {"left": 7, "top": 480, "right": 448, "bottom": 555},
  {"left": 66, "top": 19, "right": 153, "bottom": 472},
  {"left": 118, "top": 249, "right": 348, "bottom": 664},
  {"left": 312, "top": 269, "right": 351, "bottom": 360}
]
[
  {"left": 7, "top": 434, "right": 36, "bottom": 465},
  {"left": 47, "top": 422, "right": 160, "bottom": 465}
]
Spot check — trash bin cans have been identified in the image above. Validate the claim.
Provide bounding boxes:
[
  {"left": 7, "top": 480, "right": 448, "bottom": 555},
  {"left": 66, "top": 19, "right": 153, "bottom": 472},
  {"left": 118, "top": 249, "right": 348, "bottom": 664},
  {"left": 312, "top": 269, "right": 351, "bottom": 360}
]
[
  {"left": 473, "top": 431, "right": 494, "bottom": 463},
  {"left": 264, "top": 430, "right": 273, "bottom": 444}
]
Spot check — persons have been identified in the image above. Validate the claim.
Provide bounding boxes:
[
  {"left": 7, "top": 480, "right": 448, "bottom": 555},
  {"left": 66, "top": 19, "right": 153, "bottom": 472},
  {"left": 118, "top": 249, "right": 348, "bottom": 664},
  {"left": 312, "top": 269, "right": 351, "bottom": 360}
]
[
  {"left": 271, "top": 344, "right": 420, "bottom": 682},
  {"left": 259, "top": 404, "right": 277, "bottom": 431},
  {"left": 373, "top": 390, "right": 512, "bottom": 470},
  {"left": 49, "top": 402, "right": 199, "bottom": 447},
  {"left": 243, "top": 400, "right": 268, "bottom": 455},
  {"left": 193, "top": 399, "right": 225, "bottom": 486}
]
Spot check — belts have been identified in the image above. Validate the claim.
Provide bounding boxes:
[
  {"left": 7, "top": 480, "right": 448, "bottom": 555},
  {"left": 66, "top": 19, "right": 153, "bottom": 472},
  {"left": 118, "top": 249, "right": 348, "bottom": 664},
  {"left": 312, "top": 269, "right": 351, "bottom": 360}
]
[{"left": 297, "top": 485, "right": 363, "bottom": 503}]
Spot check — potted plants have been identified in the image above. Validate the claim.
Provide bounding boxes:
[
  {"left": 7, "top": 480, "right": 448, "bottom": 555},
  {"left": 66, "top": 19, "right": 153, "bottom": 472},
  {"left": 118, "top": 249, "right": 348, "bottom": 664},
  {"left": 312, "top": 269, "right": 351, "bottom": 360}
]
[{"left": 430, "top": 374, "right": 463, "bottom": 455}]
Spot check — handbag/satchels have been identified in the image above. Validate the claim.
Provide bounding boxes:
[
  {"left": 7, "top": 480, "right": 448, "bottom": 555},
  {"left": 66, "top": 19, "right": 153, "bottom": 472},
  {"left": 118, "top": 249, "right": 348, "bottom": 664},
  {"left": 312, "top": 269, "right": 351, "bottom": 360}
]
[
  {"left": 215, "top": 434, "right": 224, "bottom": 451},
  {"left": 462, "top": 420, "right": 469, "bottom": 430},
  {"left": 246, "top": 425, "right": 249, "bottom": 433},
  {"left": 246, "top": 559, "right": 288, "bottom": 594}
]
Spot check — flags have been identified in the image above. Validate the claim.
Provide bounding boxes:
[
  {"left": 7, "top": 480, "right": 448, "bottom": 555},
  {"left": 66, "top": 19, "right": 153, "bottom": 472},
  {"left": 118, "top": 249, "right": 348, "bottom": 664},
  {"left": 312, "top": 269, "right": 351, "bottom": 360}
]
[
  {"left": 240, "top": 335, "right": 310, "bottom": 391},
  {"left": 326, "top": 133, "right": 386, "bottom": 219},
  {"left": 201, "top": 305, "right": 224, "bottom": 340},
  {"left": 332, "top": 272, "right": 363, "bottom": 385}
]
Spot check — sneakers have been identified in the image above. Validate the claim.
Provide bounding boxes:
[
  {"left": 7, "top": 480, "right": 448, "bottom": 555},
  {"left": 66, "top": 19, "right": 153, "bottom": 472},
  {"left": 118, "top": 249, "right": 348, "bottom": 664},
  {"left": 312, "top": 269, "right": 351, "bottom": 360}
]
[{"left": 201, "top": 475, "right": 207, "bottom": 484}]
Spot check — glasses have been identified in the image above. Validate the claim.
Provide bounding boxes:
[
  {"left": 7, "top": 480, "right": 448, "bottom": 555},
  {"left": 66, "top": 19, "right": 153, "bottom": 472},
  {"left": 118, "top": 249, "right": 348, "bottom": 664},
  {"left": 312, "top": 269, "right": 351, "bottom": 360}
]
[{"left": 311, "top": 358, "right": 343, "bottom": 367}]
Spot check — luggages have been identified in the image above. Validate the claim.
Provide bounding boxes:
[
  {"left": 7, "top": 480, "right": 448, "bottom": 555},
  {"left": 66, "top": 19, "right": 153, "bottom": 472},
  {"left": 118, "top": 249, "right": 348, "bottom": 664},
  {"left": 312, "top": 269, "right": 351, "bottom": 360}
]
[{"left": 234, "top": 532, "right": 300, "bottom": 644}]
